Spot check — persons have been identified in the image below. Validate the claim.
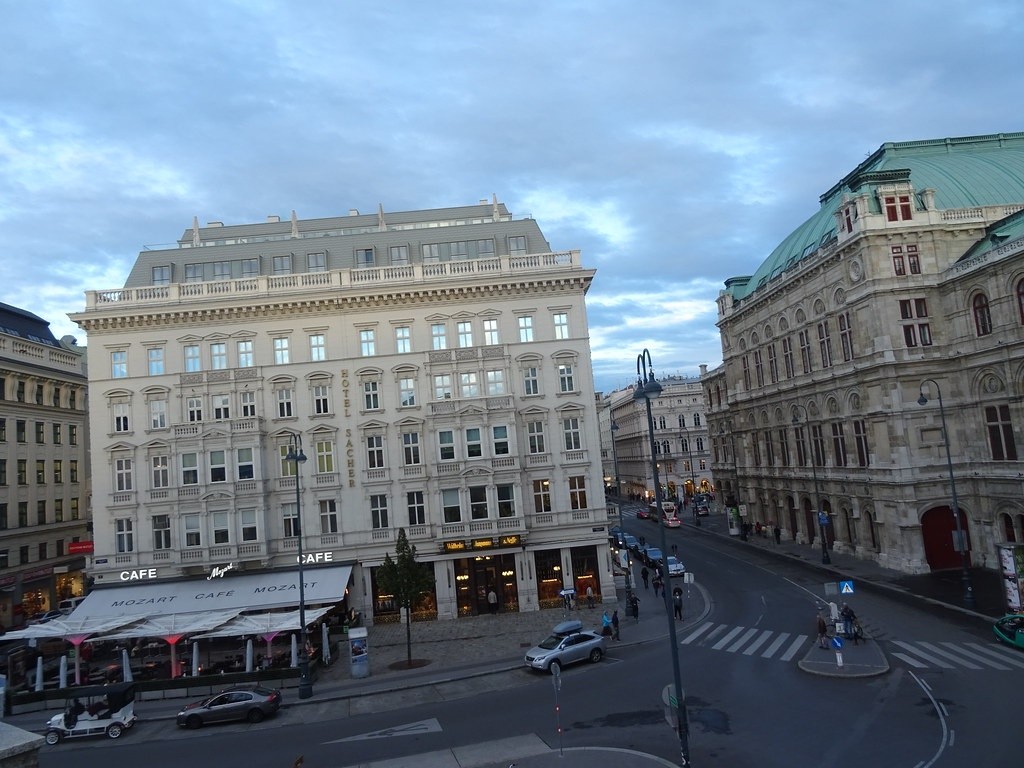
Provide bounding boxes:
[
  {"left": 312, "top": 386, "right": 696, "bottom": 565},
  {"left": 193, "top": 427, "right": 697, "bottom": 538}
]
[
  {"left": 816, "top": 615, "right": 830, "bottom": 649},
  {"left": 488, "top": 589, "right": 497, "bottom": 614},
  {"left": 840, "top": 603, "right": 858, "bottom": 639},
  {"left": 652, "top": 565, "right": 683, "bottom": 610},
  {"left": 673, "top": 592, "right": 684, "bottom": 620},
  {"left": 630, "top": 593, "right": 640, "bottom": 620},
  {"left": 641, "top": 566, "right": 649, "bottom": 589},
  {"left": 601, "top": 609, "right": 621, "bottom": 642},
  {"left": 70, "top": 699, "right": 85, "bottom": 715},
  {"left": 587, "top": 585, "right": 596, "bottom": 609},
  {"left": 629, "top": 491, "right": 716, "bottom": 513}
]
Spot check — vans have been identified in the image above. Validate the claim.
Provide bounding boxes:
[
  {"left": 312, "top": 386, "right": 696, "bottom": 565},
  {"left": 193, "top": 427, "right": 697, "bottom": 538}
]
[{"left": 57, "top": 595, "right": 88, "bottom": 615}]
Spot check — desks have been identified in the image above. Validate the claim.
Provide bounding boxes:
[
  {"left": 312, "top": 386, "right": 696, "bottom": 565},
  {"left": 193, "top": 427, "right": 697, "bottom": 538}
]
[{"left": 30, "top": 657, "right": 245, "bottom": 689}]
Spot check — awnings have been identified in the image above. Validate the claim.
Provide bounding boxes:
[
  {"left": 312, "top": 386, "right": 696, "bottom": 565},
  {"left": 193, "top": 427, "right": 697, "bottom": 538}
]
[{"left": 63, "top": 565, "right": 354, "bottom": 621}]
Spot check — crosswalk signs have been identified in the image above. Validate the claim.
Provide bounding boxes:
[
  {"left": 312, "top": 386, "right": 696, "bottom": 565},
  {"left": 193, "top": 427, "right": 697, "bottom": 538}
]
[{"left": 840, "top": 580, "right": 854, "bottom": 594}]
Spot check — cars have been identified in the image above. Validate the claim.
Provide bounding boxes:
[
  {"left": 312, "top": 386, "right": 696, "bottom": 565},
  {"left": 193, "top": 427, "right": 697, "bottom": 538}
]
[
  {"left": 662, "top": 518, "right": 680, "bottom": 528},
  {"left": 45, "top": 684, "right": 138, "bottom": 744},
  {"left": 176, "top": 684, "right": 283, "bottom": 729},
  {"left": 634, "top": 543, "right": 651, "bottom": 560},
  {"left": 650, "top": 493, "right": 716, "bottom": 519},
  {"left": 614, "top": 533, "right": 629, "bottom": 549},
  {"left": 623, "top": 536, "right": 638, "bottom": 551},
  {"left": 656, "top": 556, "right": 686, "bottom": 577},
  {"left": 25, "top": 610, "right": 65, "bottom": 628},
  {"left": 643, "top": 548, "right": 663, "bottom": 567},
  {"left": 637, "top": 508, "right": 650, "bottom": 519}
]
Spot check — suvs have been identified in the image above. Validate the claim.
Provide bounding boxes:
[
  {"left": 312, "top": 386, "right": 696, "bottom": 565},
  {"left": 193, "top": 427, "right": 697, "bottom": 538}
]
[{"left": 524, "top": 620, "right": 607, "bottom": 673}]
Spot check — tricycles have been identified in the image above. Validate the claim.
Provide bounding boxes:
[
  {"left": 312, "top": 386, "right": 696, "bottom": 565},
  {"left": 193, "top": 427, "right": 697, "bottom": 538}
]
[{"left": 992, "top": 613, "right": 1024, "bottom": 648}]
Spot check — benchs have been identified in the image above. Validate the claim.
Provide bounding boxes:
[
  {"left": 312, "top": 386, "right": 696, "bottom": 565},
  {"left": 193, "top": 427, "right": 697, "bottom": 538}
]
[{"left": 88, "top": 702, "right": 109, "bottom": 719}]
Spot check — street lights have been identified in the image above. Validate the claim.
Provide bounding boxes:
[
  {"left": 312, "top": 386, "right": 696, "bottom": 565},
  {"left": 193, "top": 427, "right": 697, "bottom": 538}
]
[
  {"left": 678, "top": 427, "right": 701, "bottom": 527},
  {"left": 633, "top": 347, "right": 691, "bottom": 767},
  {"left": 719, "top": 420, "right": 750, "bottom": 541},
  {"left": 792, "top": 405, "right": 832, "bottom": 564},
  {"left": 283, "top": 433, "right": 315, "bottom": 699},
  {"left": 609, "top": 410, "right": 636, "bottom": 616},
  {"left": 918, "top": 377, "right": 978, "bottom": 603}
]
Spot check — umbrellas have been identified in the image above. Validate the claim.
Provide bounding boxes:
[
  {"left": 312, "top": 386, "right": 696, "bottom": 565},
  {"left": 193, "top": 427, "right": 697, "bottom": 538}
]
[{"left": 0, "top": 605, "right": 336, "bottom": 642}]
